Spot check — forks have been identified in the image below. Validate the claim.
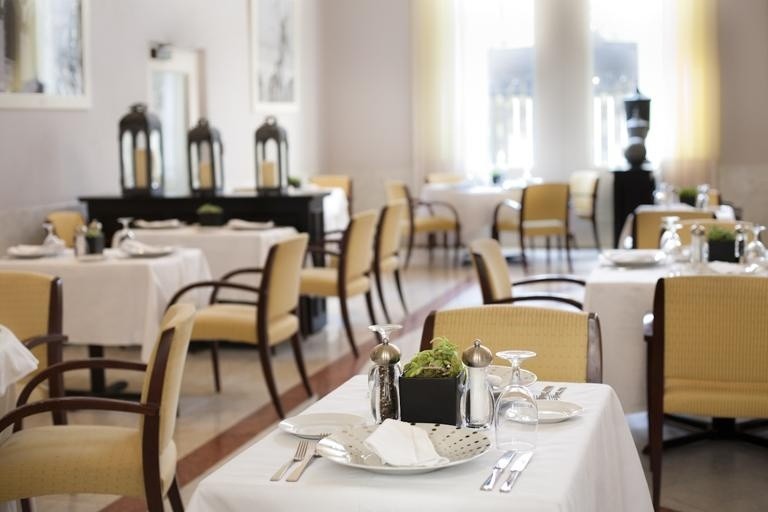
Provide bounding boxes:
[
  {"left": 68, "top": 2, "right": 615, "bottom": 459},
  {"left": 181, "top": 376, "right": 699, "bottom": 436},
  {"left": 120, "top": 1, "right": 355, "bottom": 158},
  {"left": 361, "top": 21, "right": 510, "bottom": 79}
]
[
  {"left": 548, "top": 387, "right": 567, "bottom": 400},
  {"left": 287, "top": 432, "right": 332, "bottom": 482},
  {"left": 533, "top": 386, "right": 554, "bottom": 400},
  {"left": 270, "top": 440, "right": 309, "bottom": 482}
]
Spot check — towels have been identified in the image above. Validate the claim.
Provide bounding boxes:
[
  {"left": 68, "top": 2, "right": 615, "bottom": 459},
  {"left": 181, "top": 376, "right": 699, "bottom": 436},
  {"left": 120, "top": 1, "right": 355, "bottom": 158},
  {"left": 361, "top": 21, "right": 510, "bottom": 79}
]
[{"left": 368, "top": 417, "right": 448, "bottom": 467}]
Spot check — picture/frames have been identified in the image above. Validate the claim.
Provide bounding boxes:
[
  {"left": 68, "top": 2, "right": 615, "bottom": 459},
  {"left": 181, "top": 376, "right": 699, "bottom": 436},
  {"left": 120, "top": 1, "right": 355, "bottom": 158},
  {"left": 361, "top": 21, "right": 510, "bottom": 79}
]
[
  {"left": 0, "top": 0, "right": 95, "bottom": 114},
  {"left": 251, "top": 0, "right": 301, "bottom": 115}
]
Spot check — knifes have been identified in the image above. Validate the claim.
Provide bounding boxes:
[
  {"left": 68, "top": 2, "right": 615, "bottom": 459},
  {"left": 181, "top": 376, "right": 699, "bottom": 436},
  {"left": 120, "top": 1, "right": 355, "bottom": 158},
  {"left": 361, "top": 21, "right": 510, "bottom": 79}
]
[
  {"left": 481, "top": 450, "right": 517, "bottom": 490},
  {"left": 500, "top": 451, "right": 534, "bottom": 492}
]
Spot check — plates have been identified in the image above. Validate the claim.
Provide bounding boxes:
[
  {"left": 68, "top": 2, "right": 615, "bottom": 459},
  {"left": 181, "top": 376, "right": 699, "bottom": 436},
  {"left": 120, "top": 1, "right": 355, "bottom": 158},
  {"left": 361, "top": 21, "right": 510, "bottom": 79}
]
[
  {"left": 605, "top": 247, "right": 665, "bottom": 267},
  {"left": 124, "top": 244, "right": 175, "bottom": 257},
  {"left": 487, "top": 364, "right": 537, "bottom": 398},
  {"left": 7, "top": 244, "right": 57, "bottom": 258},
  {"left": 316, "top": 423, "right": 493, "bottom": 476},
  {"left": 506, "top": 400, "right": 583, "bottom": 424},
  {"left": 279, "top": 412, "right": 366, "bottom": 440}
]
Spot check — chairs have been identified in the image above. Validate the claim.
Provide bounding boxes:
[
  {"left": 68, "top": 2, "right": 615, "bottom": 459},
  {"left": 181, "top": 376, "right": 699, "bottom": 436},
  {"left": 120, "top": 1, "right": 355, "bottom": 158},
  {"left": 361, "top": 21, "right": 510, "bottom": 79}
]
[
  {"left": 420, "top": 306, "right": 606, "bottom": 385},
  {"left": 631, "top": 205, "right": 726, "bottom": 247},
  {"left": 298, "top": 209, "right": 381, "bottom": 360},
  {"left": 159, "top": 231, "right": 313, "bottom": 420},
  {"left": 426, "top": 174, "right": 469, "bottom": 251},
  {"left": 327, "top": 204, "right": 412, "bottom": 324},
  {"left": 645, "top": 276, "right": 768, "bottom": 512},
  {"left": 384, "top": 182, "right": 460, "bottom": 269},
  {"left": 44, "top": 211, "right": 84, "bottom": 252},
  {"left": 308, "top": 174, "right": 353, "bottom": 221},
  {"left": 0, "top": 271, "right": 69, "bottom": 435},
  {"left": 671, "top": 219, "right": 757, "bottom": 248},
  {"left": 545, "top": 173, "right": 601, "bottom": 253},
  {"left": 0, "top": 300, "right": 199, "bottom": 512},
  {"left": 468, "top": 238, "right": 584, "bottom": 310},
  {"left": 704, "top": 189, "right": 747, "bottom": 220},
  {"left": 488, "top": 185, "right": 573, "bottom": 275}
]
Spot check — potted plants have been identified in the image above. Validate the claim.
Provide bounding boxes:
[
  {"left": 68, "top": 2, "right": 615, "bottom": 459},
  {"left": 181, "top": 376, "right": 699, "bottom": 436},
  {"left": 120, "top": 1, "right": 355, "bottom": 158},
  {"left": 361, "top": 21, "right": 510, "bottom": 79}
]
[
  {"left": 398, "top": 334, "right": 465, "bottom": 425},
  {"left": 193, "top": 204, "right": 224, "bottom": 228},
  {"left": 81, "top": 227, "right": 106, "bottom": 253},
  {"left": 679, "top": 189, "right": 698, "bottom": 208},
  {"left": 705, "top": 224, "right": 745, "bottom": 263}
]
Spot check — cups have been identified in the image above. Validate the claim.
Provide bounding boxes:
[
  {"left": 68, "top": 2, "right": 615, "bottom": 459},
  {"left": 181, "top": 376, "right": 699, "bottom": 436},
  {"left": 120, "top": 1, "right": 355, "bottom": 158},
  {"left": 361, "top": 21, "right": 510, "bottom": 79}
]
[
  {"left": 659, "top": 224, "right": 683, "bottom": 263},
  {"left": 367, "top": 324, "right": 403, "bottom": 398},
  {"left": 659, "top": 216, "right": 681, "bottom": 249},
  {"left": 741, "top": 227, "right": 764, "bottom": 261},
  {"left": 43, "top": 223, "right": 57, "bottom": 248},
  {"left": 117, "top": 218, "right": 135, "bottom": 245},
  {"left": 495, "top": 350, "right": 538, "bottom": 454}
]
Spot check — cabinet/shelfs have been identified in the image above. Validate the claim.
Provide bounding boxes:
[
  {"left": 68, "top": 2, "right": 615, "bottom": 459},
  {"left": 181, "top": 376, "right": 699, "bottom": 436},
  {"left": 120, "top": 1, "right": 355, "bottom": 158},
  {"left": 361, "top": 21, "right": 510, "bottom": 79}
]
[
  {"left": 78, "top": 191, "right": 330, "bottom": 336},
  {"left": 611, "top": 169, "right": 656, "bottom": 250}
]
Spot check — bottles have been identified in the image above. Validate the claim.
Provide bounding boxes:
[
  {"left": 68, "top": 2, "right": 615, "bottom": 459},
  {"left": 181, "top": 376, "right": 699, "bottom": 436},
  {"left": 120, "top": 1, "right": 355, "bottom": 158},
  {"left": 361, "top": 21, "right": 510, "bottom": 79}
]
[
  {"left": 73, "top": 224, "right": 87, "bottom": 258},
  {"left": 460, "top": 340, "right": 495, "bottom": 431},
  {"left": 733, "top": 224, "right": 749, "bottom": 259},
  {"left": 689, "top": 224, "right": 705, "bottom": 265},
  {"left": 366, "top": 338, "right": 402, "bottom": 426},
  {"left": 85, "top": 220, "right": 105, "bottom": 254}
]
